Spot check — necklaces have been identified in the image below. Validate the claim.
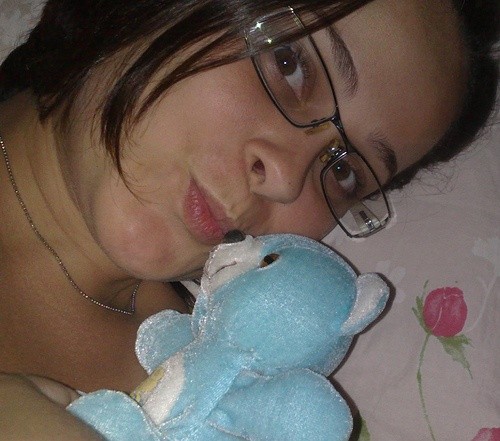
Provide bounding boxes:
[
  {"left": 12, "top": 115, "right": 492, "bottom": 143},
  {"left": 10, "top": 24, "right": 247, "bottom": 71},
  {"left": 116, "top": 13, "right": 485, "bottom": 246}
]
[{"left": 0, "top": 137, "right": 145, "bottom": 318}]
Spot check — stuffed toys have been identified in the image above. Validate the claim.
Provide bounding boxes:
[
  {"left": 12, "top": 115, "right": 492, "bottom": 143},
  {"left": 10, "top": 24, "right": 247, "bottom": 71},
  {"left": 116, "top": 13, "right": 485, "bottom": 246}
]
[{"left": 62, "top": 226, "right": 392, "bottom": 439}]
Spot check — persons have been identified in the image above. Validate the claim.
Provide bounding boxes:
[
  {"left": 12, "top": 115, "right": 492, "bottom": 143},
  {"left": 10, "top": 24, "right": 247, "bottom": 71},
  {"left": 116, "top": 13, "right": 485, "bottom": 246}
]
[{"left": 0, "top": 0, "right": 500, "bottom": 441}]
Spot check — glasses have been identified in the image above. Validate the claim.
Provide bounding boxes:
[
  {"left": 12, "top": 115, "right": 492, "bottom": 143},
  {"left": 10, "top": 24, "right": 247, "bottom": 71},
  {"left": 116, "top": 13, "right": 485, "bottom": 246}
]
[{"left": 239, "top": 6, "right": 393, "bottom": 239}]
